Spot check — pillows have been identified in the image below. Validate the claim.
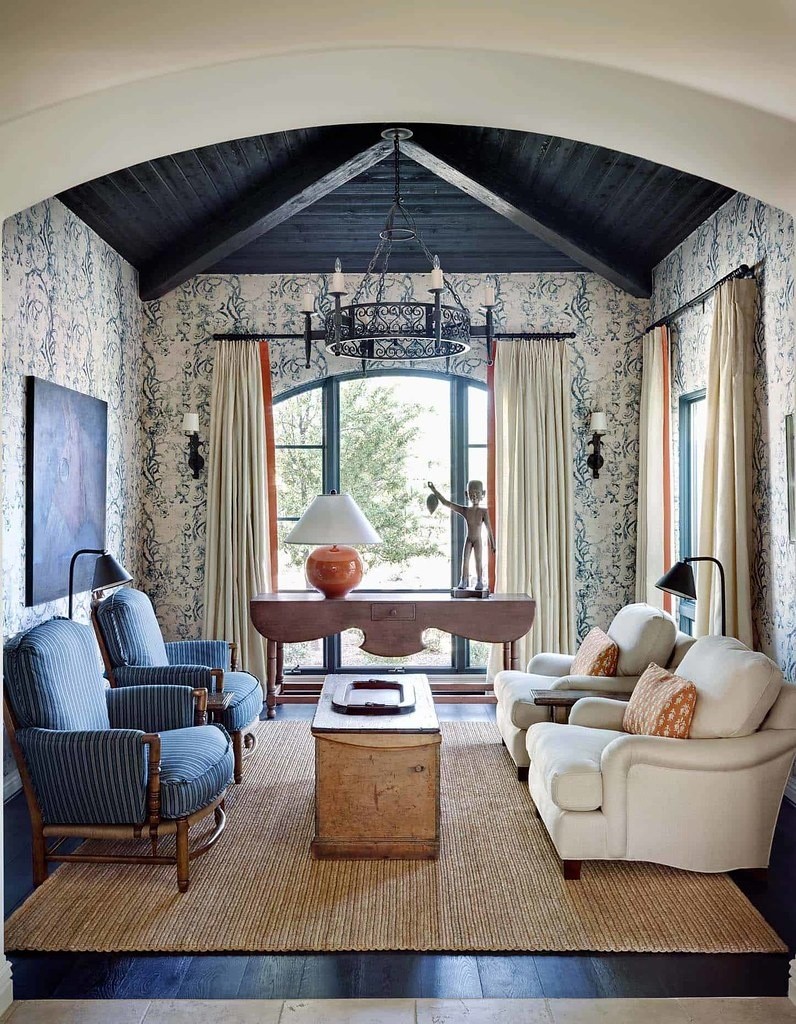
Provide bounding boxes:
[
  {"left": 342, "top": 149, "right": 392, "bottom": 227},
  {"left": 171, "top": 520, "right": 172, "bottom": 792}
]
[
  {"left": 570, "top": 626, "right": 618, "bottom": 678},
  {"left": 607, "top": 602, "right": 676, "bottom": 677},
  {"left": 674, "top": 636, "right": 783, "bottom": 739},
  {"left": 622, "top": 661, "right": 697, "bottom": 739}
]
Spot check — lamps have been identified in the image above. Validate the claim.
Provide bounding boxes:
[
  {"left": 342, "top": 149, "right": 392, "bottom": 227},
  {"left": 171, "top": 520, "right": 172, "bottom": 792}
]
[
  {"left": 282, "top": 488, "right": 384, "bottom": 600},
  {"left": 586, "top": 411, "right": 607, "bottom": 480},
  {"left": 324, "top": 131, "right": 472, "bottom": 362},
  {"left": 178, "top": 412, "right": 206, "bottom": 479},
  {"left": 68, "top": 548, "right": 134, "bottom": 619},
  {"left": 654, "top": 556, "right": 727, "bottom": 637}
]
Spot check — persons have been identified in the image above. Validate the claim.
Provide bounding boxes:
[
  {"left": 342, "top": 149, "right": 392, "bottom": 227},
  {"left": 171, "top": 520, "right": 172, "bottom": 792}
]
[{"left": 427, "top": 480, "right": 496, "bottom": 590}]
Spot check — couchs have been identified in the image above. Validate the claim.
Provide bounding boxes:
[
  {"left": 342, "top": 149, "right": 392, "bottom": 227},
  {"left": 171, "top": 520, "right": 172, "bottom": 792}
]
[
  {"left": 525, "top": 676, "right": 796, "bottom": 881},
  {"left": 493, "top": 631, "right": 695, "bottom": 783}
]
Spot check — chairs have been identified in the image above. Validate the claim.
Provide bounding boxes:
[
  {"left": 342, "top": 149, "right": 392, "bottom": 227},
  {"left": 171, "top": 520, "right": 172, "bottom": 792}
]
[
  {"left": 90, "top": 587, "right": 264, "bottom": 784},
  {"left": 4, "top": 615, "right": 235, "bottom": 893}
]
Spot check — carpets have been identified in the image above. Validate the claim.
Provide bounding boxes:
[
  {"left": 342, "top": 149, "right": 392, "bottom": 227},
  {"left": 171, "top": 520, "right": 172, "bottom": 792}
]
[{"left": 3, "top": 719, "right": 789, "bottom": 955}]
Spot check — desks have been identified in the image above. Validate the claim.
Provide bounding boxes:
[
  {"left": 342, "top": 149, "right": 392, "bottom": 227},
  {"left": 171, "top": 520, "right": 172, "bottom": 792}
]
[
  {"left": 250, "top": 592, "right": 536, "bottom": 717},
  {"left": 306, "top": 673, "right": 441, "bottom": 861},
  {"left": 195, "top": 690, "right": 234, "bottom": 711},
  {"left": 530, "top": 689, "right": 633, "bottom": 723}
]
